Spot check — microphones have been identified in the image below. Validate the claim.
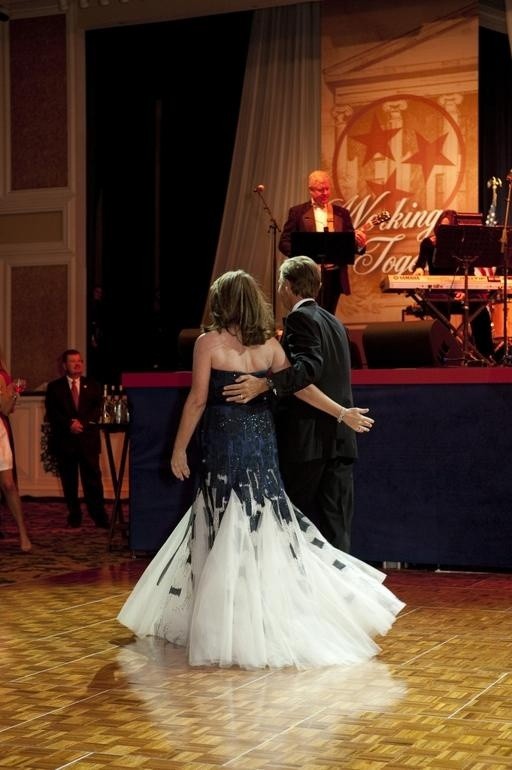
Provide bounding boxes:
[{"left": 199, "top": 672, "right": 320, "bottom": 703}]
[{"left": 254, "top": 183, "right": 265, "bottom": 193}]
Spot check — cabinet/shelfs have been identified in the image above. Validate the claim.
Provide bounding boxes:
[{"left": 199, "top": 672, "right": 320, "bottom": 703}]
[{"left": 7, "top": 396, "right": 129, "bottom": 501}]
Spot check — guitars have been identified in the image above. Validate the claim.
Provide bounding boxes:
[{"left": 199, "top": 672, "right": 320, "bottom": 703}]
[{"left": 358, "top": 211, "right": 390, "bottom": 234}]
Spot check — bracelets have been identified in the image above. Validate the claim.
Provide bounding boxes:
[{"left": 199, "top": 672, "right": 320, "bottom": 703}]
[{"left": 336, "top": 407, "right": 346, "bottom": 423}]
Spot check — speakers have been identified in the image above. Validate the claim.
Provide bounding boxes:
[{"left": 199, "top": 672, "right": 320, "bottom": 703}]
[
  {"left": 362, "top": 320, "right": 460, "bottom": 367},
  {"left": 178, "top": 329, "right": 203, "bottom": 372}
]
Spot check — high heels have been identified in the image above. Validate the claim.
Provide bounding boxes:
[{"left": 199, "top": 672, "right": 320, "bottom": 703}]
[{"left": 21, "top": 537, "right": 32, "bottom": 552}]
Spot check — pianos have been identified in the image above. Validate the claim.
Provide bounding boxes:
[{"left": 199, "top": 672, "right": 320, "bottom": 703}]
[{"left": 379, "top": 275, "right": 512, "bottom": 293}]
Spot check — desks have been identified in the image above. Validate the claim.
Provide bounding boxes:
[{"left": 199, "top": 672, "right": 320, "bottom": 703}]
[{"left": 88, "top": 422, "right": 131, "bottom": 552}]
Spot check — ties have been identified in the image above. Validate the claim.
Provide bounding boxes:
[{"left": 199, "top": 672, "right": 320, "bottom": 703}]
[{"left": 71, "top": 380, "right": 78, "bottom": 412}]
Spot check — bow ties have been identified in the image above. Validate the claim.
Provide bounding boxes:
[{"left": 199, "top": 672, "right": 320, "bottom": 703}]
[{"left": 312, "top": 202, "right": 326, "bottom": 209}]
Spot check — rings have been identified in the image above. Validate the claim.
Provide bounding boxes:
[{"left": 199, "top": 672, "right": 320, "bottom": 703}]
[{"left": 240, "top": 394, "right": 243, "bottom": 399}]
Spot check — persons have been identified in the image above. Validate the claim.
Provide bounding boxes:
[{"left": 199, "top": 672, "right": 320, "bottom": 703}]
[
  {"left": 0, "top": 370, "right": 34, "bottom": 556},
  {"left": 274, "top": 169, "right": 367, "bottom": 317},
  {"left": 164, "top": 272, "right": 377, "bottom": 669},
  {"left": 218, "top": 253, "right": 359, "bottom": 557},
  {"left": 411, "top": 211, "right": 498, "bottom": 365},
  {"left": 44, "top": 348, "right": 113, "bottom": 531}
]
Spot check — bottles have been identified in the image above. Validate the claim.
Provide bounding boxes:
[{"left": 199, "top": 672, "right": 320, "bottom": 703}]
[{"left": 102, "top": 385, "right": 129, "bottom": 424}]
